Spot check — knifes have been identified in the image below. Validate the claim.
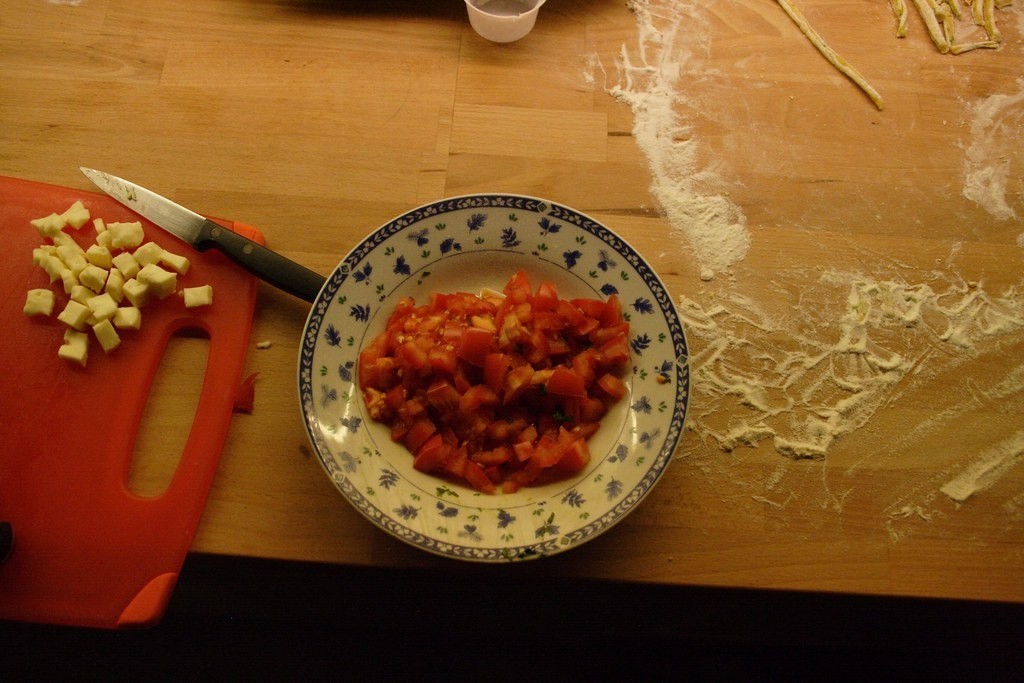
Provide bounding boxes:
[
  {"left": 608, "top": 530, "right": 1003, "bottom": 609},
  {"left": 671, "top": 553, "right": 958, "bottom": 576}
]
[{"left": 79, "top": 166, "right": 329, "bottom": 302}]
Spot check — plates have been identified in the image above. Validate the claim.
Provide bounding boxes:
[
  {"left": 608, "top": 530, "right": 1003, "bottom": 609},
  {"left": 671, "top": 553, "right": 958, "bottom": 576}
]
[{"left": 298, "top": 193, "right": 691, "bottom": 564}]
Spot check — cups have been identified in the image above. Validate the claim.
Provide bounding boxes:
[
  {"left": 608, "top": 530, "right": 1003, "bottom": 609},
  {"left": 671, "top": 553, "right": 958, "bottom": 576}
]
[{"left": 464, "top": 0, "right": 546, "bottom": 43}]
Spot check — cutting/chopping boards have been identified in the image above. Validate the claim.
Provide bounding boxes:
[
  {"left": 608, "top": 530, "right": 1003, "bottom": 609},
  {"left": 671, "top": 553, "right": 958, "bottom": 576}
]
[{"left": 0, "top": 174, "right": 266, "bottom": 631}]
